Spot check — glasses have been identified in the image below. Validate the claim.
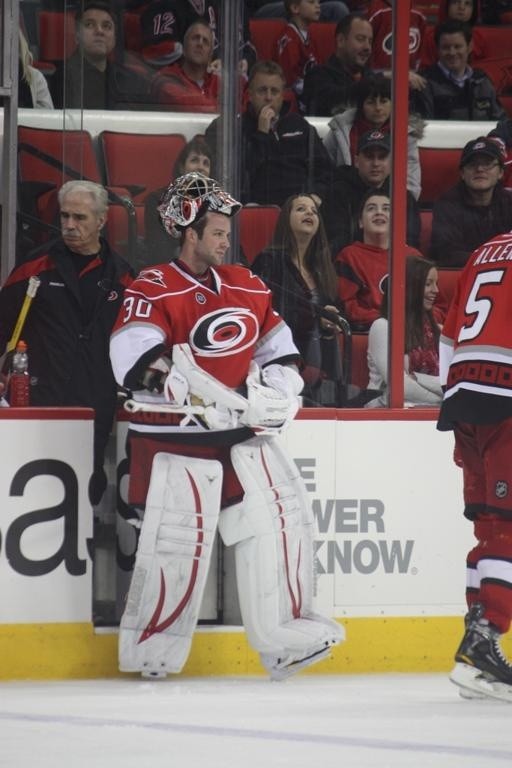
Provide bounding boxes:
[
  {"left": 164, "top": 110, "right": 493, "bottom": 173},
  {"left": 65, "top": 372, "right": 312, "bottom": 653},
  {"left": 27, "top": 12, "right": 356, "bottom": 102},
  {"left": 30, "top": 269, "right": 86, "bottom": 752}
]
[{"left": 468, "top": 158, "right": 503, "bottom": 170}]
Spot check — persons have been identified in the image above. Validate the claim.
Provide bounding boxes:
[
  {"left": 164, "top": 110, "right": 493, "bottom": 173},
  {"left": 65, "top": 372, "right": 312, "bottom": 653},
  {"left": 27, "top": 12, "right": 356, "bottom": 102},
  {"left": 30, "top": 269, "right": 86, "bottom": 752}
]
[
  {"left": 436, "top": 227, "right": 512, "bottom": 685},
  {"left": 0, "top": 0, "right": 512, "bottom": 408},
  {"left": 108, "top": 170, "right": 348, "bottom": 680}
]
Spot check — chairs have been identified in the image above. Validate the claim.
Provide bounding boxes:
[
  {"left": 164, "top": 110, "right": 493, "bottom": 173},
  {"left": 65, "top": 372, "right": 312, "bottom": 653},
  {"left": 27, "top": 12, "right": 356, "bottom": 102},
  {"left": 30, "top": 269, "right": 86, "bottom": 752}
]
[{"left": 1, "top": 1, "right": 512, "bottom": 409}]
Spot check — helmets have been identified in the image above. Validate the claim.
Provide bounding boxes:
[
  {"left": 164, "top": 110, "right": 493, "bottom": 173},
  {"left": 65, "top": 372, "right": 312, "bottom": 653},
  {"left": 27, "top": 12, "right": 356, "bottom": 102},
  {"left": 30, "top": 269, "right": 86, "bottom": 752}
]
[{"left": 158, "top": 171, "right": 241, "bottom": 239}]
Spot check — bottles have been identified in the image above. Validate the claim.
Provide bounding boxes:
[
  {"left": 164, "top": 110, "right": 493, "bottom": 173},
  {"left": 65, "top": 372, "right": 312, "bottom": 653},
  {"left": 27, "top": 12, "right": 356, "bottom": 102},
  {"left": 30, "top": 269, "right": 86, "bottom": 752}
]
[{"left": 9, "top": 342, "right": 30, "bottom": 408}]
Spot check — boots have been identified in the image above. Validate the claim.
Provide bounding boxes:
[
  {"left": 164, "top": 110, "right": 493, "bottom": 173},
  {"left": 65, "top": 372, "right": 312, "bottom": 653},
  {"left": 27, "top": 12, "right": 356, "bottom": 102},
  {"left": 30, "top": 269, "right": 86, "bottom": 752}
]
[{"left": 455, "top": 600, "right": 512, "bottom": 685}]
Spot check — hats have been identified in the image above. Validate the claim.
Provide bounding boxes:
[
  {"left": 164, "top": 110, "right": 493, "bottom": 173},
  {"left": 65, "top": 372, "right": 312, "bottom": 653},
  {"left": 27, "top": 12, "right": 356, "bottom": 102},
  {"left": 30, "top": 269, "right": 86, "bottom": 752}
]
[
  {"left": 459, "top": 137, "right": 503, "bottom": 167},
  {"left": 357, "top": 130, "right": 392, "bottom": 154}
]
